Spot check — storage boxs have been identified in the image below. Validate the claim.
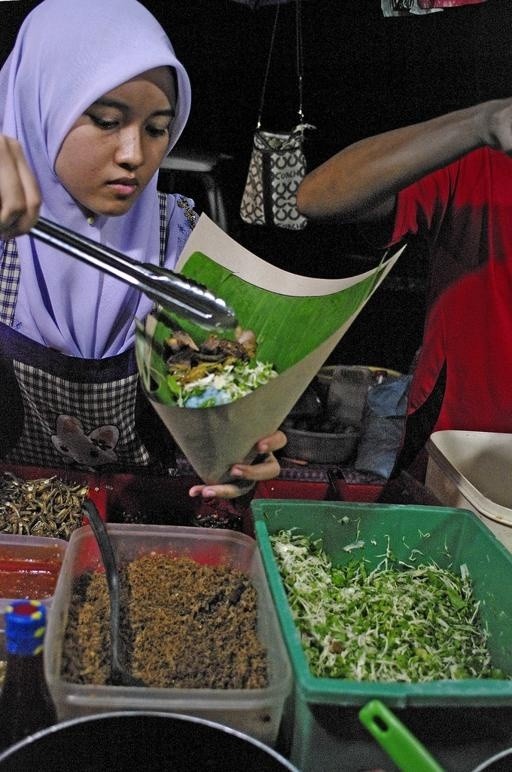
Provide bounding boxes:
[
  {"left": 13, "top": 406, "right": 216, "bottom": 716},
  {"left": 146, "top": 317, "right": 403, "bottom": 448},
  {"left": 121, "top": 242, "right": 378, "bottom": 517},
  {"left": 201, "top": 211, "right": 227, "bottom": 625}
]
[
  {"left": 1, "top": 463, "right": 94, "bottom": 541},
  {"left": 0, "top": 532, "right": 68, "bottom": 609},
  {"left": 44, "top": 522, "right": 293, "bottom": 748},
  {"left": 250, "top": 499, "right": 512, "bottom": 772},
  {"left": 426, "top": 430, "right": 511, "bottom": 550},
  {"left": 95, "top": 471, "right": 441, "bottom": 541}
]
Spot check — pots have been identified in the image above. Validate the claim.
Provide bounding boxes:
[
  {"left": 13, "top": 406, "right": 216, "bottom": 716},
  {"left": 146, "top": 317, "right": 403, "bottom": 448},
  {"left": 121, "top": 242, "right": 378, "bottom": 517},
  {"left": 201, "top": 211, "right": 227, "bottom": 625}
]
[{"left": 1, "top": 711, "right": 304, "bottom": 772}]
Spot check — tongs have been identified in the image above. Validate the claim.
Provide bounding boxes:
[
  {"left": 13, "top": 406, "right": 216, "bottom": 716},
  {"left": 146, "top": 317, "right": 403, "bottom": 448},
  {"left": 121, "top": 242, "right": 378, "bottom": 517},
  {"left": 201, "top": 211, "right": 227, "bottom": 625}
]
[{"left": 24, "top": 212, "right": 239, "bottom": 335}]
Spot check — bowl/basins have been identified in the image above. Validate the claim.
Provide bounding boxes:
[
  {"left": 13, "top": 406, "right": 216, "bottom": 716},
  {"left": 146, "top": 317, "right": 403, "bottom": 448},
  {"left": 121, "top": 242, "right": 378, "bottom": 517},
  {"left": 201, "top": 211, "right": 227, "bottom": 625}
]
[{"left": 273, "top": 365, "right": 404, "bottom": 463}]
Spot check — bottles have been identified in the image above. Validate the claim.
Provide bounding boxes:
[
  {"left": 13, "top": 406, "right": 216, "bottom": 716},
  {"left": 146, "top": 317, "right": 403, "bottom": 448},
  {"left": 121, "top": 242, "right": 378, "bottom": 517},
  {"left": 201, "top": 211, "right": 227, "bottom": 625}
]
[{"left": 2, "top": 602, "right": 66, "bottom": 748}]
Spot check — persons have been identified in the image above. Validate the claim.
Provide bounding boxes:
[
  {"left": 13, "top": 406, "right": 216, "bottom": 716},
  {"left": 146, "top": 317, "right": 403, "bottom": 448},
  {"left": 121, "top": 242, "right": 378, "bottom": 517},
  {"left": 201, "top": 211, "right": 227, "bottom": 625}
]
[
  {"left": 295, "top": 95, "right": 511, "bottom": 479},
  {"left": 0, "top": 0, "right": 287, "bottom": 498}
]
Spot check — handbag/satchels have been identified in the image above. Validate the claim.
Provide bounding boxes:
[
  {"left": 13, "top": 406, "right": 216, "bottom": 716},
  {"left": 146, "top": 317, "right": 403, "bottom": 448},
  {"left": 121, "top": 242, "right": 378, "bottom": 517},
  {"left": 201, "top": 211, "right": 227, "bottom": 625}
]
[{"left": 237, "top": 122, "right": 317, "bottom": 232}]
